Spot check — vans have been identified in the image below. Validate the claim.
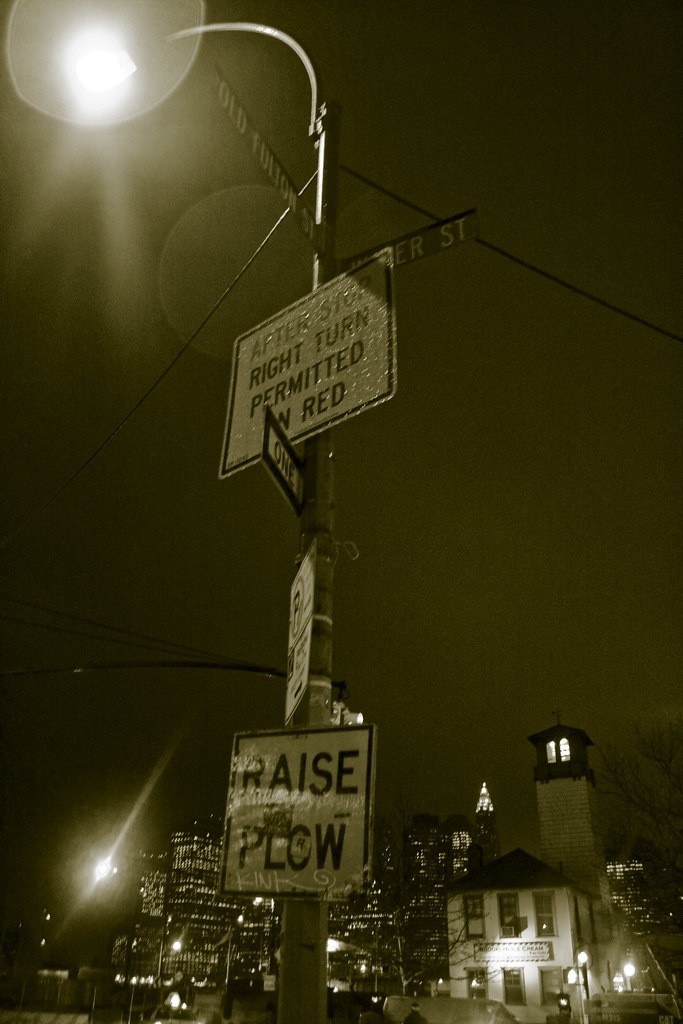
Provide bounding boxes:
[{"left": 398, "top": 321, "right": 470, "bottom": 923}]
[
  {"left": 589, "top": 993, "right": 683, "bottom": 1024},
  {"left": 383, "top": 994, "right": 523, "bottom": 1024}
]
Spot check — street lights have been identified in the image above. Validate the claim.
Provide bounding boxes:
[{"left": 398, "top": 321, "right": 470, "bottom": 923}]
[
  {"left": 73, "top": 22, "right": 336, "bottom": 1023},
  {"left": 625, "top": 961, "right": 635, "bottom": 994},
  {"left": 580, "top": 950, "right": 590, "bottom": 1002}
]
